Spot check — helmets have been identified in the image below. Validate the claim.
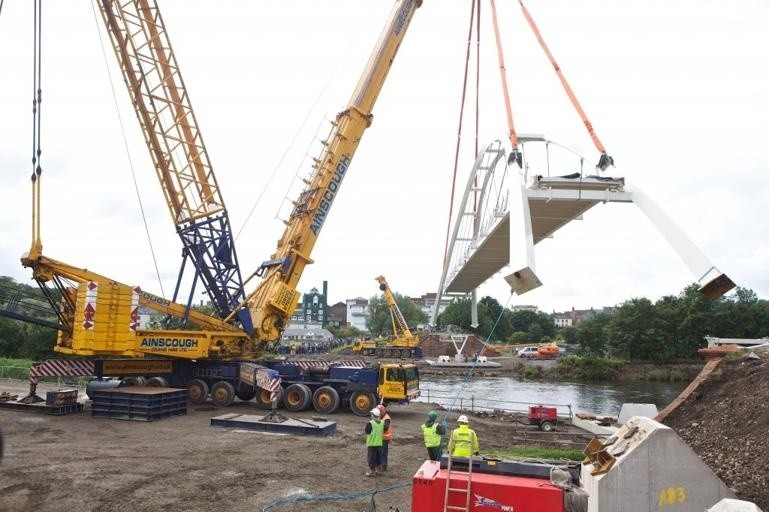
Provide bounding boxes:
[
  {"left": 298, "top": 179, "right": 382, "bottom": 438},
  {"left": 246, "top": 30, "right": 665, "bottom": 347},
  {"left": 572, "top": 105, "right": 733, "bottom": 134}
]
[
  {"left": 370, "top": 409, "right": 381, "bottom": 417},
  {"left": 457, "top": 415, "right": 469, "bottom": 423},
  {"left": 429, "top": 411, "right": 437, "bottom": 421}
]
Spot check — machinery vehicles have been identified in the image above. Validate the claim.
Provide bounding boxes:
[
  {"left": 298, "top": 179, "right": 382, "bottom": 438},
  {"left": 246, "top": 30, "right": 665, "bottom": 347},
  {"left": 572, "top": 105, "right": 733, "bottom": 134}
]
[
  {"left": 347, "top": 274, "right": 423, "bottom": 359},
  {"left": 0, "top": 0, "right": 614, "bottom": 417}
]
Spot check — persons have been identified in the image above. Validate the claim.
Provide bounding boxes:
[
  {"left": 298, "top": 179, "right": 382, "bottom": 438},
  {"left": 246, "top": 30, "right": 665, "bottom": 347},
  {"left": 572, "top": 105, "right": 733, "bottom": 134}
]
[
  {"left": 447, "top": 415, "right": 480, "bottom": 458},
  {"left": 512, "top": 347, "right": 518, "bottom": 354},
  {"left": 364, "top": 408, "right": 384, "bottom": 476},
  {"left": 463, "top": 351, "right": 479, "bottom": 363},
  {"left": 420, "top": 411, "right": 446, "bottom": 460},
  {"left": 262, "top": 334, "right": 370, "bottom": 355},
  {"left": 376, "top": 405, "right": 392, "bottom": 470}
]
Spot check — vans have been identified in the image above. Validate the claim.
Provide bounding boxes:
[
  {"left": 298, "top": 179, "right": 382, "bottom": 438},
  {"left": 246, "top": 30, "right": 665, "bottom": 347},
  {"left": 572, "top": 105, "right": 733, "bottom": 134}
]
[{"left": 517, "top": 346, "right": 539, "bottom": 358}]
